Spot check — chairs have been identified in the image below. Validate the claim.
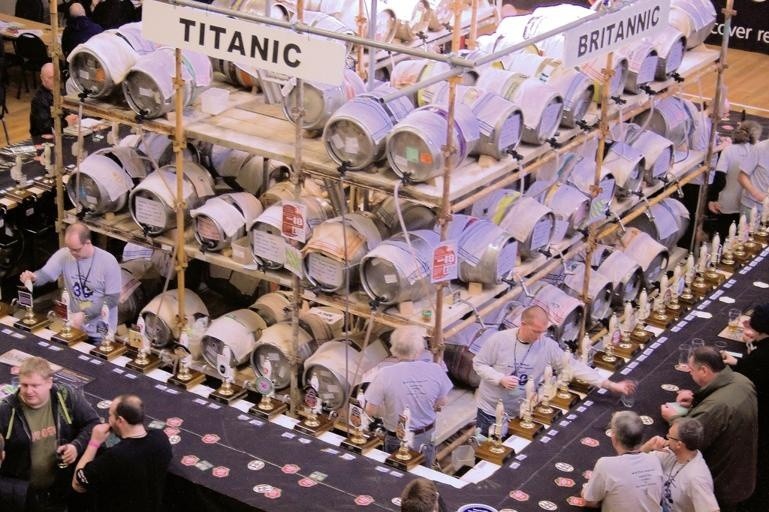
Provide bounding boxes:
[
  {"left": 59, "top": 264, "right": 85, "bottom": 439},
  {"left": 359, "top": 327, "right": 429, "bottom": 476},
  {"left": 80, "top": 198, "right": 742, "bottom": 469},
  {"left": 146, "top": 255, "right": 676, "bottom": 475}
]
[{"left": 1, "top": 1, "right": 54, "bottom": 100}]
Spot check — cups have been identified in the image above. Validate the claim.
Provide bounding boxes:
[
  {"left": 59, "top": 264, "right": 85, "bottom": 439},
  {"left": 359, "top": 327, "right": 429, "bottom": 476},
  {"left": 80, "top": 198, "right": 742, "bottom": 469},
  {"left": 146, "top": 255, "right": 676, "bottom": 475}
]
[
  {"left": 677, "top": 338, "right": 705, "bottom": 366},
  {"left": 727, "top": 308, "right": 741, "bottom": 333},
  {"left": 622, "top": 395, "right": 637, "bottom": 407},
  {"left": 91, "top": 125, "right": 101, "bottom": 143},
  {"left": 714, "top": 340, "right": 727, "bottom": 359}
]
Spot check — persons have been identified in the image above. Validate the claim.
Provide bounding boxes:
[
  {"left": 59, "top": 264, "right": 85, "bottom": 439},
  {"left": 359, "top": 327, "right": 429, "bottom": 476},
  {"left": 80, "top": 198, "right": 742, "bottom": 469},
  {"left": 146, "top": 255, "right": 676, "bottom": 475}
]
[
  {"left": 719, "top": 301, "right": 768, "bottom": 504},
  {"left": 400, "top": 478, "right": 446, "bottom": 512},
  {"left": 19, "top": 222, "right": 121, "bottom": 339},
  {"left": 707, "top": 119, "right": 763, "bottom": 247},
  {"left": 580, "top": 409, "right": 665, "bottom": 512},
  {"left": 72, "top": 393, "right": 172, "bottom": 511},
  {"left": 678, "top": 93, "right": 730, "bottom": 247},
  {"left": 660, "top": 346, "right": 759, "bottom": 512},
  {"left": 29, "top": 61, "right": 79, "bottom": 135},
  {"left": 473, "top": 303, "right": 639, "bottom": 442},
  {"left": 60, "top": 0, "right": 144, "bottom": 61},
  {"left": 0, "top": 356, "right": 101, "bottom": 511},
  {"left": 640, "top": 416, "right": 721, "bottom": 512},
  {"left": 363, "top": 324, "right": 454, "bottom": 469},
  {"left": 737, "top": 134, "right": 769, "bottom": 224}
]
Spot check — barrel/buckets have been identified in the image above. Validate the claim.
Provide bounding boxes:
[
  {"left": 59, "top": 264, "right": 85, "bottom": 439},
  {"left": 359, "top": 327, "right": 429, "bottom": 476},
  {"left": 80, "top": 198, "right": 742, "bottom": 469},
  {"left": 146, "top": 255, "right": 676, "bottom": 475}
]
[{"left": 58, "top": 1, "right": 722, "bottom": 414}]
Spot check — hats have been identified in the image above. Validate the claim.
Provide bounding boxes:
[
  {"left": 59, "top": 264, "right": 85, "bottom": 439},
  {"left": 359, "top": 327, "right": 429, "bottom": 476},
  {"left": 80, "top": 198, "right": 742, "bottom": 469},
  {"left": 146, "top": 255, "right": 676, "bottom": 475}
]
[{"left": 749, "top": 305, "right": 768, "bottom": 333}]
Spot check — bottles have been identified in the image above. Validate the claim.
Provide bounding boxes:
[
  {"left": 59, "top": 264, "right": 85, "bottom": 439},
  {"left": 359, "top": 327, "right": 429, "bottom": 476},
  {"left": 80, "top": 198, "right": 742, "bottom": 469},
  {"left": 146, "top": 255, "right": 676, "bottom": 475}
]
[{"left": 421, "top": 308, "right": 432, "bottom": 324}]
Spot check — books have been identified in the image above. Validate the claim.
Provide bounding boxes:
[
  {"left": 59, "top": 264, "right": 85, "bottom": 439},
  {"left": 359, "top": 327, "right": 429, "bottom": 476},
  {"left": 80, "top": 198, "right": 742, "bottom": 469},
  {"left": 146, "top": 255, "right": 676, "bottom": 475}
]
[
  {"left": 81, "top": 117, "right": 109, "bottom": 132},
  {"left": 63, "top": 123, "right": 92, "bottom": 138},
  {"left": 11, "top": 145, "right": 37, "bottom": 154}
]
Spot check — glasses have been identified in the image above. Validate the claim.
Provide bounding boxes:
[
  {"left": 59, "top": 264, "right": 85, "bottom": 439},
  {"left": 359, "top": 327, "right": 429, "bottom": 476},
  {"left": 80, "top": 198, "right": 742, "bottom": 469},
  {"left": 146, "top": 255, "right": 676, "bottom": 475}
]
[{"left": 666, "top": 434, "right": 682, "bottom": 443}]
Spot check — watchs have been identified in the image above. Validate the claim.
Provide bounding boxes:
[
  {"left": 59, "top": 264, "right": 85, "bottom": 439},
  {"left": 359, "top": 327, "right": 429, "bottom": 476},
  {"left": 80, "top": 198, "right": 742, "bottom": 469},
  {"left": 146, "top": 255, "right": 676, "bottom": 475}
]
[{"left": 82, "top": 310, "right": 90, "bottom": 325}]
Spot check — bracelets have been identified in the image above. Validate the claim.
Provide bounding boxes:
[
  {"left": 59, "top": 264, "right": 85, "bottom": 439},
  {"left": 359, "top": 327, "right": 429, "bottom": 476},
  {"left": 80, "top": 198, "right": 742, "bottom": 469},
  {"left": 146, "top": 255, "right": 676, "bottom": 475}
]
[
  {"left": 608, "top": 381, "right": 615, "bottom": 389},
  {"left": 88, "top": 441, "right": 100, "bottom": 449}
]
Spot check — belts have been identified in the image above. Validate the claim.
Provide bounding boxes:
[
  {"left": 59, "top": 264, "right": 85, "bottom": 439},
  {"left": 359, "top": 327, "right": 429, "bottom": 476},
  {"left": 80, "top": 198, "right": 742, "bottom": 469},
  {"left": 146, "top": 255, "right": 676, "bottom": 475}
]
[{"left": 389, "top": 425, "right": 433, "bottom": 437}]
